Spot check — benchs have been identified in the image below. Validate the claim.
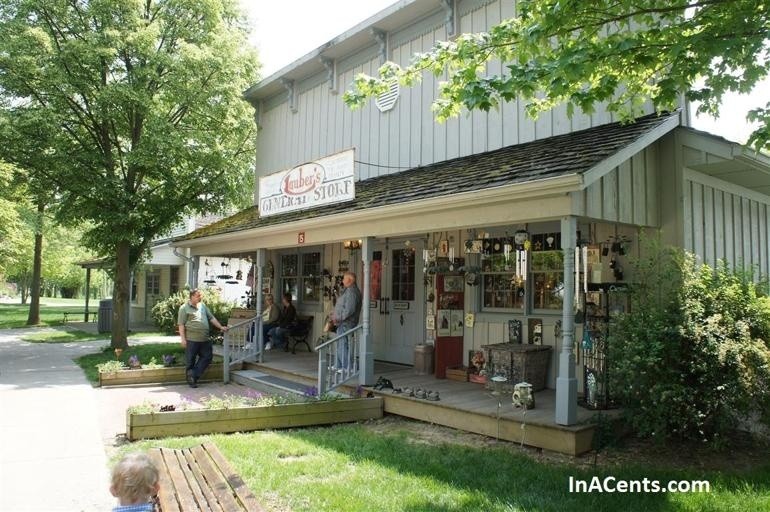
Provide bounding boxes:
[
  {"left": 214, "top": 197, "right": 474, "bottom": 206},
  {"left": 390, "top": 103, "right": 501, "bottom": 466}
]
[
  {"left": 148, "top": 440, "right": 263, "bottom": 512},
  {"left": 284, "top": 315, "right": 314, "bottom": 354},
  {"left": 63, "top": 312, "right": 98, "bottom": 323}
]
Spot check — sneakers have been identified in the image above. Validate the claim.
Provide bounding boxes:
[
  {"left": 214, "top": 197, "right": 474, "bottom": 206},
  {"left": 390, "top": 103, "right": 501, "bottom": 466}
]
[
  {"left": 242, "top": 342, "right": 253, "bottom": 349},
  {"left": 327, "top": 366, "right": 353, "bottom": 374},
  {"left": 186, "top": 368, "right": 198, "bottom": 387},
  {"left": 265, "top": 341, "right": 272, "bottom": 350}
]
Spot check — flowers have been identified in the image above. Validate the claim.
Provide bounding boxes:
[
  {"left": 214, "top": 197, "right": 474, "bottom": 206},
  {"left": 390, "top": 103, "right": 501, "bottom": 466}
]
[{"left": 344, "top": 240, "right": 362, "bottom": 249}]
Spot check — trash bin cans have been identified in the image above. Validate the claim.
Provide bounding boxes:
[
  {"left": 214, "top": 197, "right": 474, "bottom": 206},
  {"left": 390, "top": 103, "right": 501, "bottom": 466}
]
[
  {"left": 414, "top": 343, "right": 434, "bottom": 376},
  {"left": 97, "top": 299, "right": 113, "bottom": 333}
]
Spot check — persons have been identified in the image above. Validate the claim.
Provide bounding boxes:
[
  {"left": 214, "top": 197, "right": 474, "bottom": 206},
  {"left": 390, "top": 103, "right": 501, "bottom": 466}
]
[
  {"left": 176, "top": 288, "right": 230, "bottom": 388},
  {"left": 327, "top": 270, "right": 362, "bottom": 374},
  {"left": 240, "top": 293, "right": 282, "bottom": 351},
  {"left": 271, "top": 293, "right": 299, "bottom": 351},
  {"left": 108, "top": 452, "right": 161, "bottom": 512}
]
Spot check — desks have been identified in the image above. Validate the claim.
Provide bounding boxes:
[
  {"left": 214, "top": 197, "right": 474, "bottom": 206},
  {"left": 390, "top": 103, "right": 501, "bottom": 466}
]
[{"left": 481, "top": 343, "right": 552, "bottom": 392}]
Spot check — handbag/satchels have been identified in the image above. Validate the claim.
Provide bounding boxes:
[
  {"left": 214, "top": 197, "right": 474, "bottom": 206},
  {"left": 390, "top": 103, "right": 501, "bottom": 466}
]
[{"left": 323, "top": 313, "right": 338, "bottom": 334}]
